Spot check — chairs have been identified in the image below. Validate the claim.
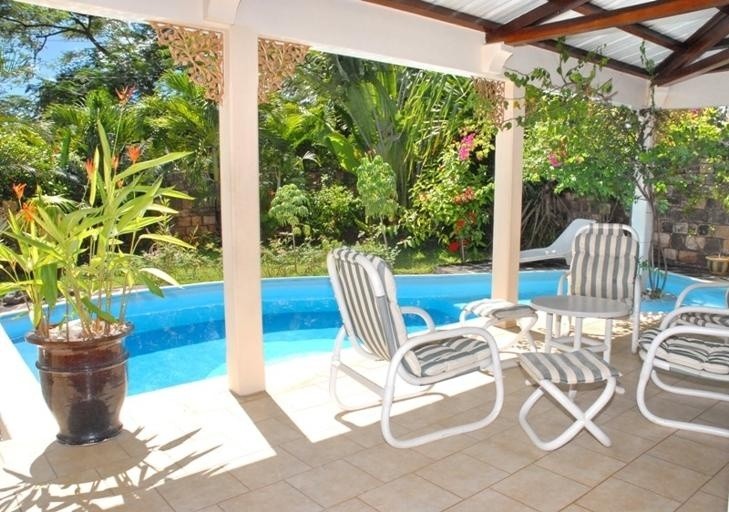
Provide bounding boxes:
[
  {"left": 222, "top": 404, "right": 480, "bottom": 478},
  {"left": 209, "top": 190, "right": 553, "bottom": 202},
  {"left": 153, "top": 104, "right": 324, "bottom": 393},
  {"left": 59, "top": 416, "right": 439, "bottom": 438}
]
[
  {"left": 636, "top": 281, "right": 729, "bottom": 437},
  {"left": 327, "top": 247, "right": 504, "bottom": 452},
  {"left": 556, "top": 221, "right": 643, "bottom": 352},
  {"left": 521, "top": 216, "right": 595, "bottom": 266}
]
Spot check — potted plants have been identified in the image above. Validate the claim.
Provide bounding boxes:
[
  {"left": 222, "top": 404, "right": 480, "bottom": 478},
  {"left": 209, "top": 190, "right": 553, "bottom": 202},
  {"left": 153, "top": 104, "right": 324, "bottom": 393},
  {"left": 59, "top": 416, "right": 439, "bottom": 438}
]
[
  {"left": 0, "top": 83, "right": 197, "bottom": 448},
  {"left": 705, "top": 252, "right": 728, "bottom": 276}
]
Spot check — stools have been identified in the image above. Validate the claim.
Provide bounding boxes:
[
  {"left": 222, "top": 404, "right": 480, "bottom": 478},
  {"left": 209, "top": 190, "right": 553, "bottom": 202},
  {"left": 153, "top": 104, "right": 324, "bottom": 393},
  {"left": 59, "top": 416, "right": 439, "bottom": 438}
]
[
  {"left": 452, "top": 298, "right": 625, "bottom": 452},
  {"left": 459, "top": 297, "right": 538, "bottom": 370},
  {"left": 518, "top": 352, "right": 624, "bottom": 452}
]
